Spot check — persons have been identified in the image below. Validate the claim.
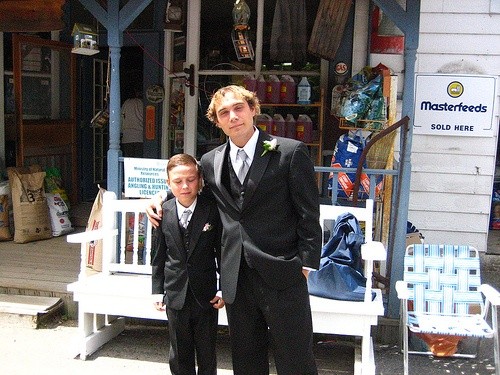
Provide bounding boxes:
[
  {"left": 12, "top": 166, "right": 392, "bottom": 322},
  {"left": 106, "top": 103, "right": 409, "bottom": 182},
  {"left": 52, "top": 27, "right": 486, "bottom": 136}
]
[
  {"left": 146, "top": 85, "right": 322, "bottom": 375},
  {"left": 121, "top": 86, "right": 145, "bottom": 157},
  {"left": 150, "top": 154, "right": 224, "bottom": 375}
]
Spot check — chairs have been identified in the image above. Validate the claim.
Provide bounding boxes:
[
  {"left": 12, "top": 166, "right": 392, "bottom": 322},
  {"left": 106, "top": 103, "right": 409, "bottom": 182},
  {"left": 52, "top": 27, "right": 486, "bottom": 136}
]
[{"left": 394, "top": 243, "right": 500, "bottom": 374}]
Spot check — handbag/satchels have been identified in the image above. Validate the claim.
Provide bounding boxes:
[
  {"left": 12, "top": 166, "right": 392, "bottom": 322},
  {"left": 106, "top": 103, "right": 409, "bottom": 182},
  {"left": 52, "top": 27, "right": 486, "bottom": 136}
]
[{"left": 308, "top": 211, "right": 377, "bottom": 303}]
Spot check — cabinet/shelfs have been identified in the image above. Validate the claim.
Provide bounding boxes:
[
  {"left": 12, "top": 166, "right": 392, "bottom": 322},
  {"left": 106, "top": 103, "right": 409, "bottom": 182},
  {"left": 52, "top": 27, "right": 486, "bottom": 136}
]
[{"left": 231, "top": 88, "right": 326, "bottom": 193}]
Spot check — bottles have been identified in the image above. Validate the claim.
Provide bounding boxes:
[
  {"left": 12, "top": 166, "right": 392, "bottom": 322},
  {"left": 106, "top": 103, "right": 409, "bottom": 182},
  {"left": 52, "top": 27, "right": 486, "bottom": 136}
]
[
  {"left": 285, "top": 114, "right": 296, "bottom": 140},
  {"left": 296, "top": 76, "right": 311, "bottom": 105},
  {"left": 244, "top": 72, "right": 256, "bottom": 93},
  {"left": 255, "top": 113, "right": 272, "bottom": 135},
  {"left": 280, "top": 74, "right": 295, "bottom": 103},
  {"left": 265, "top": 74, "right": 279, "bottom": 104},
  {"left": 297, "top": 114, "right": 312, "bottom": 143},
  {"left": 272, "top": 113, "right": 284, "bottom": 137},
  {"left": 254, "top": 73, "right": 265, "bottom": 104}
]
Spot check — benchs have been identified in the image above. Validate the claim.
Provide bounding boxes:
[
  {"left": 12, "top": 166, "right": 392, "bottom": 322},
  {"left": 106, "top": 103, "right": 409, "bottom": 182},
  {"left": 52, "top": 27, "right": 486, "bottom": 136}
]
[{"left": 66, "top": 188, "right": 389, "bottom": 375}]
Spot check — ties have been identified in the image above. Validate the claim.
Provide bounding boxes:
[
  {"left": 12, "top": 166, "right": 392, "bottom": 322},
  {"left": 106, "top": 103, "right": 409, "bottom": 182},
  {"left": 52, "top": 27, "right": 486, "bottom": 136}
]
[
  {"left": 180, "top": 209, "right": 192, "bottom": 229},
  {"left": 236, "top": 149, "right": 250, "bottom": 185}
]
